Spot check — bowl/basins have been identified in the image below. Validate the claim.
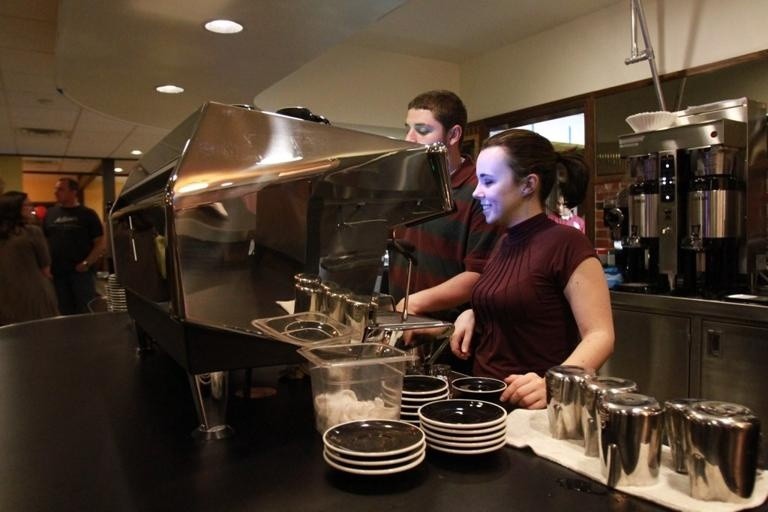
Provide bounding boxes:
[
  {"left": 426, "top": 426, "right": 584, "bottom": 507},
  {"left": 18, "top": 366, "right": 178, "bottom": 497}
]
[{"left": 284, "top": 320, "right": 341, "bottom": 345}]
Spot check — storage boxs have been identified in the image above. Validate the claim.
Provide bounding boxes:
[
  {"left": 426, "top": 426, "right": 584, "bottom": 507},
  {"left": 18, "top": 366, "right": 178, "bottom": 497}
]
[{"left": 248, "top": 310, "right": 421, "bottom": 439}]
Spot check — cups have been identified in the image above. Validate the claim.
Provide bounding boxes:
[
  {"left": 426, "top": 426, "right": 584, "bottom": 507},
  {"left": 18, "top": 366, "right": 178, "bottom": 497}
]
[
  {"left": 294, "top": 274, "right": 371, "bottom": 344},
  {"left": 545, "top": 364, "right": 760, "bottom": 504}
]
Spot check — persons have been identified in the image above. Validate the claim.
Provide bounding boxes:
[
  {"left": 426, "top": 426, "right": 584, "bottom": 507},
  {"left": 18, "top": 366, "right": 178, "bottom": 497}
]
[
  {"left": 0, "top": 177, "right": 106, "bottom": 328},
  {"left": 448, "top": 128, "right": 615, "bottom": 410},
  {"left": 388, "top": 91, "right": 506, "bottom": 377},
  {"left": 151, "top": 229, "right": 169, "bottom": 281}
]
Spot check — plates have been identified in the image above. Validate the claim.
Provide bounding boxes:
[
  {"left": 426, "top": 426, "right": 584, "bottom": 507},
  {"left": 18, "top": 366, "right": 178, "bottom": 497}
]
[
  {"left": 106, "top": 273, "right": 130, "bottom": 314},
  {"left": 318, "top": 375, "right": 508, "bottom": 483}
]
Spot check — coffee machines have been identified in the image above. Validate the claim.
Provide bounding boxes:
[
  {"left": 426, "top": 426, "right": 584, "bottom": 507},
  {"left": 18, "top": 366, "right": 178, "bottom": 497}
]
[{"left": 603, "top": 97, "right": 768, "bottom": 291}]
